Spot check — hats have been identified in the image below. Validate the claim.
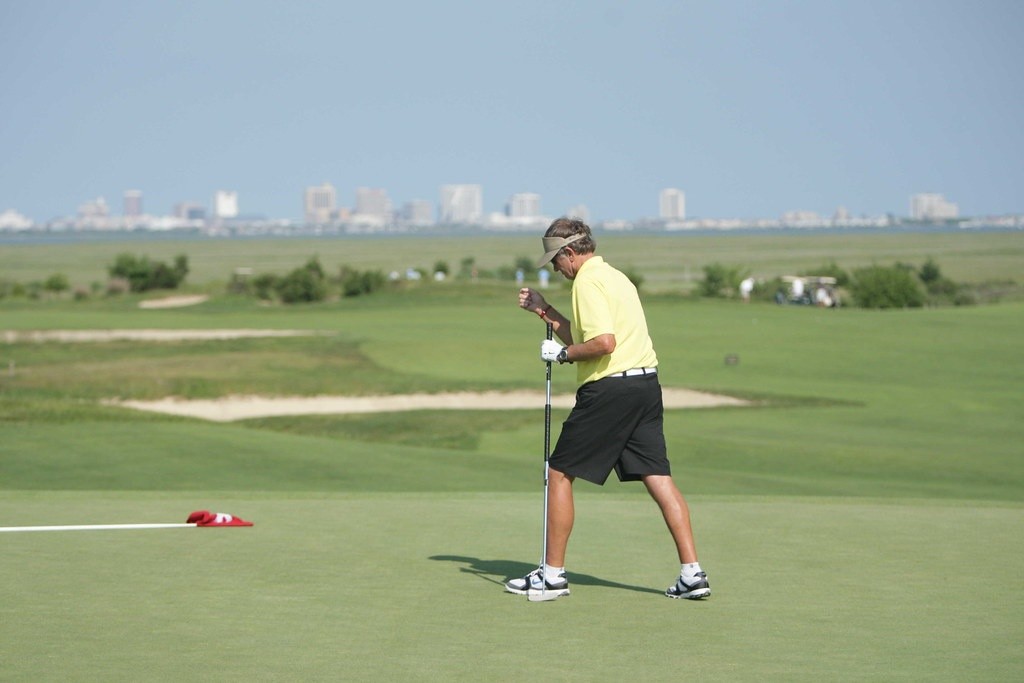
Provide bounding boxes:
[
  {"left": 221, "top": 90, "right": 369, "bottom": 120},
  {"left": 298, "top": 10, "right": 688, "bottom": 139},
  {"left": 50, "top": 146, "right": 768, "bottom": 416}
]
[{"left": 535, "top": 232, "right": 587, "bottom": 270}]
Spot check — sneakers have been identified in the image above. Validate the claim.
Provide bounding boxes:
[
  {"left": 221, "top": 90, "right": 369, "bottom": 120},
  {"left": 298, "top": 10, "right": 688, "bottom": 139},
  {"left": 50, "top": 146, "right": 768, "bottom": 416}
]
[
  {"left": 506, "top": 567, "right": 570, "bottom": 597},
  {"left": 664, "top": 571, "right": 712, "bottom": 599}
]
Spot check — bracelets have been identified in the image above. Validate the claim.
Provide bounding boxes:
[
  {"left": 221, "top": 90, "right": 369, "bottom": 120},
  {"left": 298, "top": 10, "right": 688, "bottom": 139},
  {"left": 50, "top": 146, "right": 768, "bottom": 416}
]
[{"left": 540, "top": 305, "right": 552, "bottom": 318}]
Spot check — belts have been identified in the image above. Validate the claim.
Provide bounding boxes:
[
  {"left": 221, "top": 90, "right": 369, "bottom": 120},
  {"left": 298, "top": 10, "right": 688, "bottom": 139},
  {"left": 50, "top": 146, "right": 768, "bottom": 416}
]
[{"left": 607, "top": 367, "right": 657, "bottom": 377}]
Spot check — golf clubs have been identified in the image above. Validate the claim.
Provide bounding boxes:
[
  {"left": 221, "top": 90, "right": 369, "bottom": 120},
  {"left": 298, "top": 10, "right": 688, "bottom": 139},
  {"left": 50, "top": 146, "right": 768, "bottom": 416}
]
[{"left": 528, "top": 322, "right": 557, "bottom": 601}]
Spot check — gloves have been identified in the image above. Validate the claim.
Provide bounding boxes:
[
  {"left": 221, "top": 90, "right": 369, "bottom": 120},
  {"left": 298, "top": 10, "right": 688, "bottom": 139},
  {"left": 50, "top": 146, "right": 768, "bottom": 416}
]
[{"left": 541, "top": 337, "right": 564, "bottom": 364}]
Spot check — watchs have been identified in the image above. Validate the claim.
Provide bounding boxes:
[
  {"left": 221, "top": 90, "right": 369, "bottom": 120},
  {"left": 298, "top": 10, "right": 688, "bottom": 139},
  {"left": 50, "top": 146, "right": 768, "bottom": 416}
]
[{"left": 558, "top": 346, "right": 574, "bottom": 364}]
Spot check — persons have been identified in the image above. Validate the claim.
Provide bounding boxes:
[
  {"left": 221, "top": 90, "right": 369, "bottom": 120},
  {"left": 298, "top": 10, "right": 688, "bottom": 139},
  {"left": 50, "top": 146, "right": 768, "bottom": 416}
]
[{"left": 504, "top": 216, "right": 711, "bottom": 599}]
